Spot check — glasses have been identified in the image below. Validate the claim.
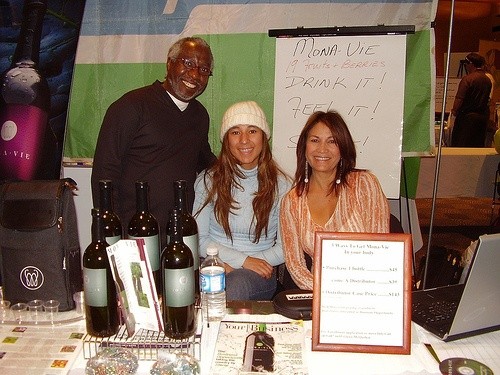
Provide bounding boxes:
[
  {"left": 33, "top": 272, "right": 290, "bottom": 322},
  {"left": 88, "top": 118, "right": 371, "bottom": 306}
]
[{"left": 175, "top": 57, "right": 212, "bottom": 76}]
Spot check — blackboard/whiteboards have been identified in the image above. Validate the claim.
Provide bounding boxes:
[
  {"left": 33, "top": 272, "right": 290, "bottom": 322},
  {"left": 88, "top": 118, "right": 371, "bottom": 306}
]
[{"left": 267, "top": 24, "right": 406, "bottom": 199}]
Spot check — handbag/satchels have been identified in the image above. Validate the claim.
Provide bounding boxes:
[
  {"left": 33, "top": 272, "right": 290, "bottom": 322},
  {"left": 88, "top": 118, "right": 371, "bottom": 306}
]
[
  {"left": 0, "top": 178, "right": 85, "bottom": 312},
  {"left": 416, "top": 245, "right": 463, "bottom": 290}
]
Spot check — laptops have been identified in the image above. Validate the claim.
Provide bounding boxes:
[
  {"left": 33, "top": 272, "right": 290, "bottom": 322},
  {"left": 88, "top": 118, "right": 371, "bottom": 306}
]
[{"left": 410, "top": 233, "right": 500, "bottom": 343}]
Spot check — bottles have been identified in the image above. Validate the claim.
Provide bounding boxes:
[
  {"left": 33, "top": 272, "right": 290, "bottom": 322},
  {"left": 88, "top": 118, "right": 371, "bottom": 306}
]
[
  {"left": 83, "top": 208, "right": 120, "bottom": 337},
  {"left": 198, "top": 246, "right": 226, "bottom": 322},
  {"left": 128, "top": 179, "right": 161, "bottom": 300},
  {"left": 159, "top": 208, "right": 199, "bottom": 339},
  {"left": 111, "top": 255, "right": 130, "bottom": 317},
  {"left": 91, "top": 181, "right": 124, "bottom": 246},
  {"left": 165, "top": 180, "right": 200, "bottom": 310},
  {"left": 242, "top": 322, "right": 275, "bottom": 371},
  {"left": 0, "top": 0, "right": 48, "bottom": 183}
]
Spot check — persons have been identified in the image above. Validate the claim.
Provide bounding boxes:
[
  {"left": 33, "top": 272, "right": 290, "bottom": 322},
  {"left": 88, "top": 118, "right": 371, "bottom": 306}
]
[
  {"left": 90, "top": 37, "right": 220, "bottom": 301},
  {"left": 447, "top": 53, "right": 494, "bottom": 148},
  {"left": 193, "top": 102, "right": 392, "bottom": 302}
]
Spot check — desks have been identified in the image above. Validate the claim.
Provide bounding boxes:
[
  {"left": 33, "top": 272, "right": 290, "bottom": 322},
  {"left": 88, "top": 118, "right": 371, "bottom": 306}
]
[{"left": 0, "top": 300, "right": 500, "bottom": 375}]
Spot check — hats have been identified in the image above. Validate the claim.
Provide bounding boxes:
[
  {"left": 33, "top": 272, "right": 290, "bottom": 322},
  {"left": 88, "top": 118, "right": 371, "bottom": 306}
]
[
  {"left": 220, "top": 101, "right": 271, "bottom": 144},
  {"left": 465, "top": 53, "right": 487, "bottom": 67}
]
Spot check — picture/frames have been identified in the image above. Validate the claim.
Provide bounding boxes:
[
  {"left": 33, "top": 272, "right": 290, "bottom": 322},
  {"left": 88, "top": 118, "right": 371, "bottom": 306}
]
[{"left": 312, "top": 231, "right": 412, "bottom": 355}]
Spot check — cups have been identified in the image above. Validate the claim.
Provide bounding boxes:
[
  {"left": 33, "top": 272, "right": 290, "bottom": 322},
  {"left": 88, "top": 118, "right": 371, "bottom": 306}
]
[
  {"left": 0, "top": 286, "right": 3, "bottom": 301},
  {"left": 0, "top": 300, "right": 11, "bottom": 320},
  {"left": 42, "top": 300, "right": 60, "bottom": 320},
  {"left": 72, "top": 291, "right": 84, "bottom": 314},
  {"left": 10, "top": 302, "right": 28, "bottom": 321},
  {"left": 26, "top": 299, "right": 44, "bottom": 319}
]
